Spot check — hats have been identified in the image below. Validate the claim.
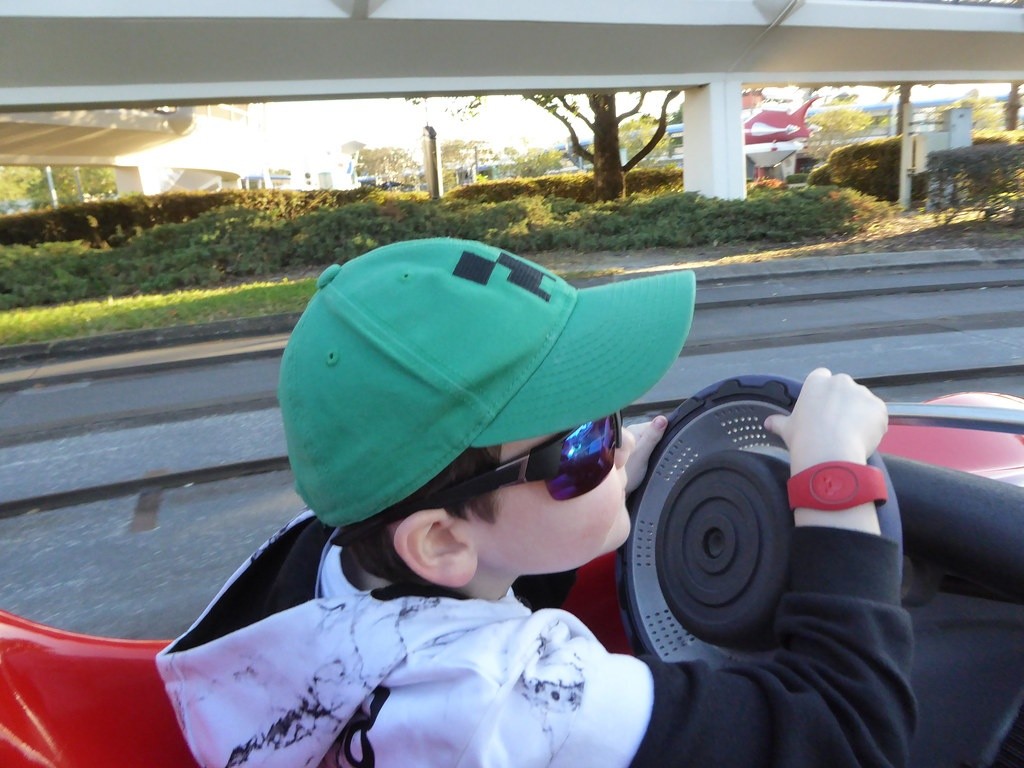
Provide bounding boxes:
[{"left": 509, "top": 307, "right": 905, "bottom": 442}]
[{"left": 277, "top": 236, "right": 698, "bottom": 530}]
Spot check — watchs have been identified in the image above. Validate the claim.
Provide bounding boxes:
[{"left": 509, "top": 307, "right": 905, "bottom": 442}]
[{"left": 785, "top": 458, "right": 888, "bottom": 511}]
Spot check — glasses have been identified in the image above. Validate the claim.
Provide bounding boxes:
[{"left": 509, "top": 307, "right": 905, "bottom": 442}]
[{"left": 330, "top": 408, "right": 623, "bottom": 548}]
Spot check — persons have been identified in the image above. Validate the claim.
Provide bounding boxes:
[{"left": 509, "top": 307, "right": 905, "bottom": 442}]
[{"left": 152, "top": 236, "right": 921, "bottom": 768}]
